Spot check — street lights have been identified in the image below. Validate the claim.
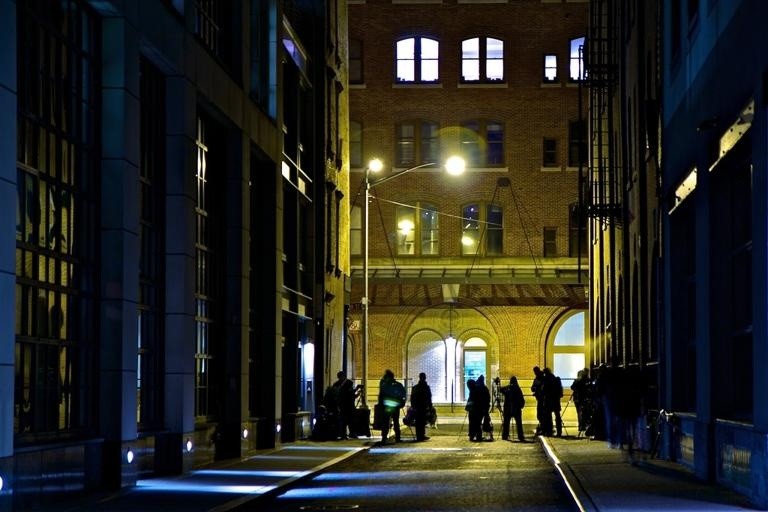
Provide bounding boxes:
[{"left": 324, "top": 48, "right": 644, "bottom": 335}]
[{"left": 348, "top": 149, "right": 470, "bottom": 440}]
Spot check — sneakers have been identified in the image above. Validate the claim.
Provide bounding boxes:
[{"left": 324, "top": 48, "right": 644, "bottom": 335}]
[
  {"left": 470, "top": 436, "right": 493, "bottom": 441},
  {"left": 536, "top": 430, "right": 561, "bottom": 437},
  {"left": 502, "top": 436, "right": 507, "bottom": 440},
  {"left": 609, "top": 442, "right": 624, "bottom": 450},
  {"left": 519, "top": 436, "right": 525, "bottom": 440},
  {"left": 417, "top": 435, "right": 430, "bottom": 441},
  {"left": 382, "top": 439, "right": 402, "bottom": 444}
]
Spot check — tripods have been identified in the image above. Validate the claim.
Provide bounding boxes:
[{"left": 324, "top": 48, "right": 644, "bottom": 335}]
[{"left": 355, "top": 385, "right": 574, "bottom": 442}]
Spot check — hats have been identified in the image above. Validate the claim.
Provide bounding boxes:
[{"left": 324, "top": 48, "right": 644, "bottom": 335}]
[
  {"left": 419, "top": 373, "right": 426, "bottom": 380},
  {"left": 338, "top": 371, "right": 346, "bottom": 378},
  {"left": 386, "top": 370, "right": 393, "bottom": 377},
  {"left": 477, "top": 375, "right": 484, "bottom": 383},
  {"left": 510, "top": 376, "right": 517, "bottom": 384}
]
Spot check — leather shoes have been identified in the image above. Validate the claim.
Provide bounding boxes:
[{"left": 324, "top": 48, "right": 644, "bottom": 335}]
[
  {"left": 349, "top": 433, "right": 357, "bottom": 438},
  {"left": 590, "top": 436, "right": 604, "bottom": 440}
]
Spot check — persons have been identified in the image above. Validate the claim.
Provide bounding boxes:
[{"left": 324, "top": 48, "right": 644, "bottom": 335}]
[
  {"left": 499, "top": 376, "right": 525, "bottom": 441},
  {"left": 570, "top": 367, "right": 589, "bottom": 431},
  {"left": 543, "top": 368, "right": 563, "bottom": 436},
  {"left": 531, "top": 366, "right": 551, "bottom": 436},
  {"left": 601, "top": 356, "right": 628, "bottom": 450},
  {"left": 491, "top": 376, "right": 502, "bottom": 407},
  {"left": 410, "top": 371, "right": 433, "bottom": 441},
  {"left": 466, "top": 375, "right": 490, "bottom": 440},
  {"left": 378, "top": 369, "right": 406, "bottom": 443},
  {"left": 332, "top": 370, "right": 357, "bottom": 439}
]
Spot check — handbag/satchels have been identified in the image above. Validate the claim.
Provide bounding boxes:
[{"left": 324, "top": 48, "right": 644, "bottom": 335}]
[
  {"left": 428, "top": 406, "right": 436, "bottom": 424},
  {"left": 403, "top": 407, "right": 415, "bottom": 427},
  {"left": 483, "top": 416, "right": 493, "bottom": 432}
]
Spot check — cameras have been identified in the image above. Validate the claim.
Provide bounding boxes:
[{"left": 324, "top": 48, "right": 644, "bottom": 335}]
[{"left": 494, "top": 377, "right": 500, "bottom": 385}]
